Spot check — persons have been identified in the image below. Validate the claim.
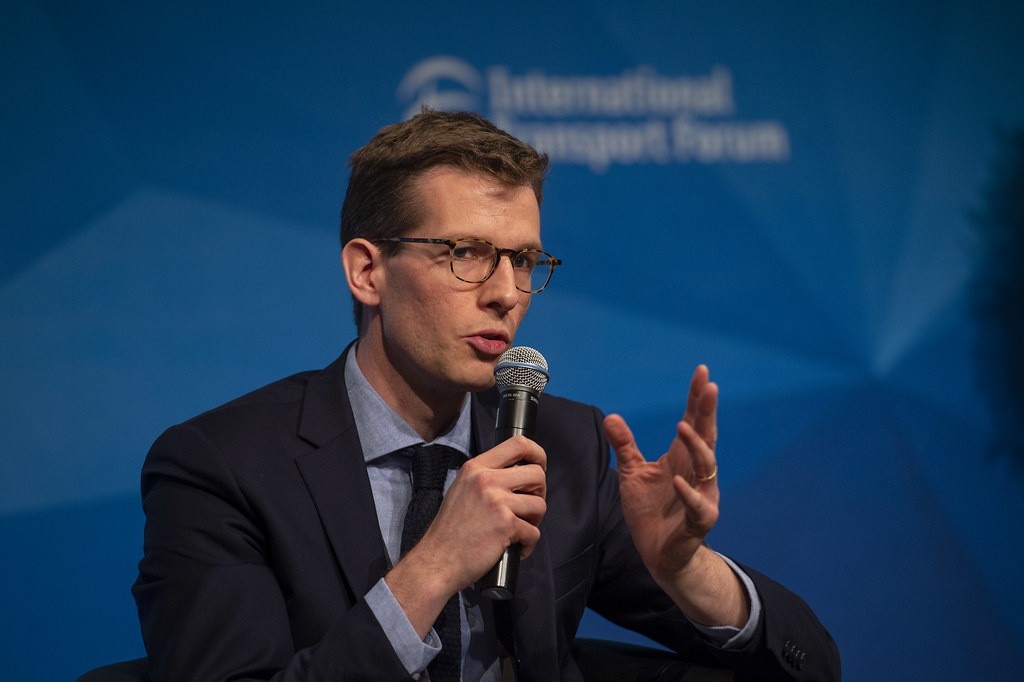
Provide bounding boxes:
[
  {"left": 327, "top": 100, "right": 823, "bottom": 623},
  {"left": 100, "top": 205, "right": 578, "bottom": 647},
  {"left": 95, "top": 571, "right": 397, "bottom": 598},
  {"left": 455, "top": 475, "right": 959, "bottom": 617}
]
[{"left": 131, "top": 106, "right": 843, "bottom": 682}]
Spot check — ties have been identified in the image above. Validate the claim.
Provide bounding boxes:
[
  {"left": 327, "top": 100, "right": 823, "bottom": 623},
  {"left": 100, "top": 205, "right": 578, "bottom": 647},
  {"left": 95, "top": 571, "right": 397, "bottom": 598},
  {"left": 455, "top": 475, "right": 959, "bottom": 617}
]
[{"left": 391, "top": 444, "right": 467, "bottom": 682}]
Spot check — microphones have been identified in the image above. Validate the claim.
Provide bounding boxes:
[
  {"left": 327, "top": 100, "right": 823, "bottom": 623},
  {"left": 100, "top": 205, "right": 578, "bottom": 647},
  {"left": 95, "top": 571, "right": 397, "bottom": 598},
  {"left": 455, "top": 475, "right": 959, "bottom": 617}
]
[{"left": 476, "top": 347, "right": 550, "bottom": 603}]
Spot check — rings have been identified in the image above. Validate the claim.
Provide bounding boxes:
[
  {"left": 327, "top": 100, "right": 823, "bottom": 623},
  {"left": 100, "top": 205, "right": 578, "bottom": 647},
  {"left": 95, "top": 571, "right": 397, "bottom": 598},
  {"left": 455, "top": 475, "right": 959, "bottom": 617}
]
[{"left": 696, "top": 466, "right": 718, "bottom": 482}]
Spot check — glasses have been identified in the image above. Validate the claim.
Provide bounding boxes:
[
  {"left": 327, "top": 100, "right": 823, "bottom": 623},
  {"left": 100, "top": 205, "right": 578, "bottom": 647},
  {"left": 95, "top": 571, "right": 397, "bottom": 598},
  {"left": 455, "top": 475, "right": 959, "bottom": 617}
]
[{"left": 368, "top": 237, "right": 563, "bottom": 295}]
